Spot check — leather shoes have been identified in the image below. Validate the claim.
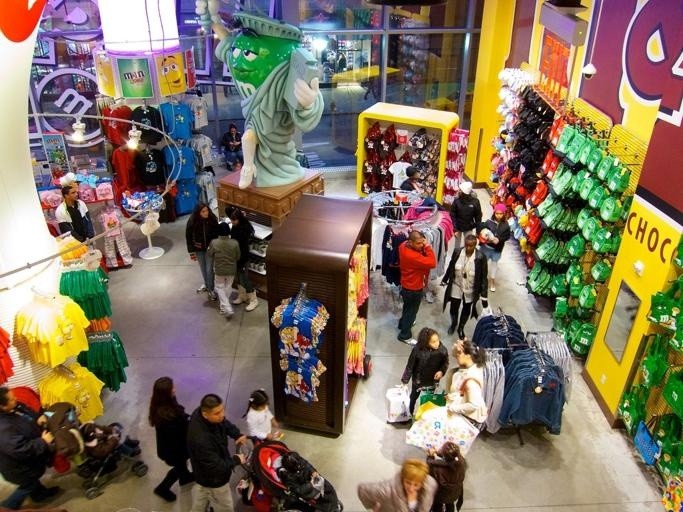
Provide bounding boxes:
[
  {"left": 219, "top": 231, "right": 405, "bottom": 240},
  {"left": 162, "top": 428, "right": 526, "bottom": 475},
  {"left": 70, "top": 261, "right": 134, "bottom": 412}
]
[
  {"left": 457, "top": 328, "right": 465, "bottom": 340},
  {"left": 448, "top": 322, "right": 457, "bottom": 335}
]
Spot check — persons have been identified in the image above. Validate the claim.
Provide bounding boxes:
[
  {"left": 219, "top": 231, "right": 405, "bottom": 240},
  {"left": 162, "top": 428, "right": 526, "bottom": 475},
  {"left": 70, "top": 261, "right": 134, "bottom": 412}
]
[
  {"left": 478, "top": 204, "right": 510, "bottom": 292},
  {"left": 243, "top": 390, "right": 279, "bottom": 441},
  {"left": 400, "top": 327, "right": 449, "bottom": 417},
  {"left": 426, "top": 441, "right": 466, "bottom": 512},
  {"left": 400, "top": 166, "right": 420, "bottom": 191},
  {"left": 439, "top": 234, "right": 488, "bottom": 340},
  {"left": 0, "top": 388, "right": 142, "bottom": 511},
  {"left": 444, "top": 340, "right": 488, "bottom": 431},
  {"left": 449, "top": 181, "right": 482, "bottom": 249},
  {"left": 280, "top": 452, "right": 333, "bottom": 508},
  {"left": 148, "top": 376, "right": 195, "bottom": 503},
  {"left": 357, "top": 459, "right": 439, "bottom": 512},
  {"left": 397, "top": 230, "right": 437, "bottom": 346},
  {"left": 185, "top": 201, "right": 259, "bottom": 319},
  {"left": 220, "top": 123, "right": 244, "bottom": 172},
  {"left": 55, "top": 186, "right": 94, "bottom": 243},
  {"left": 186, "top": 394, "right": 248, "bottom": 512}
]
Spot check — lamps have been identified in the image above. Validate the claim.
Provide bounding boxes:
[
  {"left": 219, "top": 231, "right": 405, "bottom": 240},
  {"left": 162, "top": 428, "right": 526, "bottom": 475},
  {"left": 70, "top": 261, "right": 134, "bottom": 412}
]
[
  {"left": 126, "top": 124, "right": 142, "bottom": 148},
  {"left": 70, "top": 117, "right": 88, "bottom": 142},
  {"left": 617, "top": 280, "right": 683, "bottom": 512},
  {"left": 144, "top": 209, "right": 160, "bottom": 235}
]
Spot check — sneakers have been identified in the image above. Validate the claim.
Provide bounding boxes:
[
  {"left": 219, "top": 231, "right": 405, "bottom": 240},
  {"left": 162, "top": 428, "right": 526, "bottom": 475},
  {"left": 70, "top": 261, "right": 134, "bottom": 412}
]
[
  {"left": 33, "top": 437, "right": 196, "bottom": 503},
  {"left": 398, "top": 334, "right": 417, "bottom": 346},
  {"left": 197, "top": 285, "right": 259, "bottom": 318}
]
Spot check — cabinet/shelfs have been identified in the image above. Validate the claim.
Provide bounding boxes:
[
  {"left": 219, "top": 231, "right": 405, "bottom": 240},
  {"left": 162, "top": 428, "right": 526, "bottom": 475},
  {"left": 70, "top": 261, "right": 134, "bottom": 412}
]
[
  {"left": 266, "top": 192, "right": 373, "bottom": 436},
  {"left": 218, "top": 166, "right": 325, "bottom": 302},
  {"left": 478, "top": 311, "right": 563, "bottom": 374},
  {"left": 332, "top": 65, "right": 405, "bottom": 151},
  {"left": 487, "top": 65, "right": 637, "bottom": 355},
  {"left": 357, "top": 101, "right": 460, "bottom": 206}
]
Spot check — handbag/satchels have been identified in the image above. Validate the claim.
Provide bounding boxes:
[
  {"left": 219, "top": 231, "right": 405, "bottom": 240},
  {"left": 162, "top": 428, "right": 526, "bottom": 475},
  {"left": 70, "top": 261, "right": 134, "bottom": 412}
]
[
  {"left": 617, "top": 237, "right": 683, "bottom": 512},
  {"left": 452, "top": 395, "right": 488, "bottom": 423}
]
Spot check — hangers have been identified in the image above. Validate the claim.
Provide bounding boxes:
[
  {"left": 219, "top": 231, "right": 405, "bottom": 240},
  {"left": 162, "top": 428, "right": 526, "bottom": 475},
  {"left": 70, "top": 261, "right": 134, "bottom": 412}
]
[
  {"left": 96, "top": 95, "right": 125, "bottom": 110},
  {"left": 30, "top": 229, "right": 114, "bottom": 384},
  {"left": 185, "top": 89, "right": 198, "bottom": 100},
  {"left": 369, "top": 192, "right": 444, "bottom": 234},
  {"left": 142, "top": 95, "right": 178, "bottom": 112},
  {"left": 290, "top": 290, "right": 308, "bottom": 324}
]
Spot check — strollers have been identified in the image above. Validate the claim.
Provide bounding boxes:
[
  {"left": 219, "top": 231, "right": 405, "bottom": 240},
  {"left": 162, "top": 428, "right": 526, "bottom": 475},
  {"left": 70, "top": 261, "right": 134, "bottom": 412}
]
[
  {"left": 235, "top": 433, "right": 343, "bottom": 511},
  {"left": 42, "top": 406, "right": 148, "bottom": 499}
]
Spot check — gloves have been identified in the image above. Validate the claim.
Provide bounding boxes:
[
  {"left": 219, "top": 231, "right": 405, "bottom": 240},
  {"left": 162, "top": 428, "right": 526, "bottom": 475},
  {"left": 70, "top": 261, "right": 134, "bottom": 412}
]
[
  {"left": 190, "top": 254, "right": 197, "bottom": 261},
  {"left": 482, "top": 300, "right": 488, "bottom": 308}
]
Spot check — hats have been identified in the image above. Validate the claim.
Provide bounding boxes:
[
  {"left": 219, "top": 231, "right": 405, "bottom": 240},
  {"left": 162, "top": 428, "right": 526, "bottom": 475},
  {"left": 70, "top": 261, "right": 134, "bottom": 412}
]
[
  {"left": 494, "top": 203, "right": 507, "bottom": 214},
  {"left": 459, "top": 182, "right": 473, "bottom": 195}
]
